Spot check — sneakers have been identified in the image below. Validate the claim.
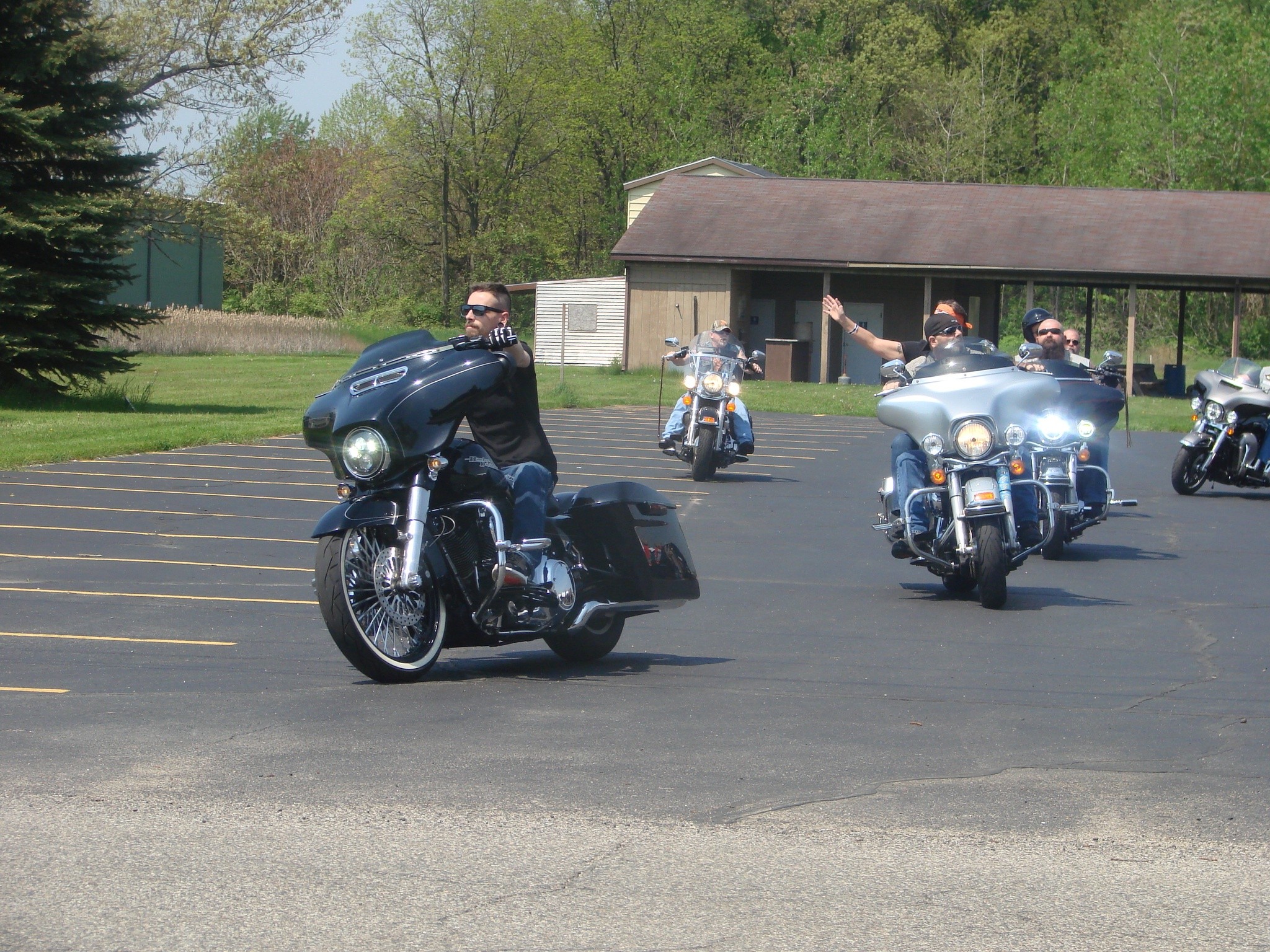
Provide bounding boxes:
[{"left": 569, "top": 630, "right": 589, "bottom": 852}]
[{"left": 492, "top": 552, "right": 533, "bottom": 586}]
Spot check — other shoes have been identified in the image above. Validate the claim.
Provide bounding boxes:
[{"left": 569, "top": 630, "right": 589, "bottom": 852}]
[
  {"left": 1016, "top": 521, "right": 1043, "bottom": 547},
  {"left": 1247, "top": 462, "right": 1265, "bottom": 478},
  {"left": 892, "top": 534, "right": 933, "bottom": 559},
  {"left": 735, "top": 441, "right": 754, "bottom": 456},
  {"left": 1082, "top": 503, "right": 1107, "bottom": 521},
  {"left": 659, "top": 438, "right": 676, "bottom": 448}
]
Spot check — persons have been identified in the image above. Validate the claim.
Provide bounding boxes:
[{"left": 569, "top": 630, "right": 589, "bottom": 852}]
[
  {"left": 459, "top": 282, "right": 559, "bottom": 585},
  {"left": 1236, "top": 368, "right": 1270, "bottom": 478},
  {"left": 658, "top": 320, "right": 762, "bottom": 454},
  {"left": 817, "top": 294, "right": 1044, "bottom": 559},
  {"left": 1017, "top": 309, "right": 1118, "bottom": 520}
]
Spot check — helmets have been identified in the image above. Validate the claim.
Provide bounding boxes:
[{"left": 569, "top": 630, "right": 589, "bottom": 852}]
[{"left": 1022, "top": 308, "right": 1054, "bottom": 343}]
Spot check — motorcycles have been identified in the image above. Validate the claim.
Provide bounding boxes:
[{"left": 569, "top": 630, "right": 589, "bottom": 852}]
[
  {"left": 1171, "top": 356, "right": 1270, "bottom": 496},
  {"left": 300, "top": 328, "right": 702, "bottom": 683},
  {"left": 657, "top": 336, "right": 766, "bottom": 482},
  {"left": 872, "top": 334, "right": 1139, "bottom": 610}
]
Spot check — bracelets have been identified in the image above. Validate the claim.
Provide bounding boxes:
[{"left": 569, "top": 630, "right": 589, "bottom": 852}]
[{"left": 847, "top": 323, "right": 858, "bottom": 335}]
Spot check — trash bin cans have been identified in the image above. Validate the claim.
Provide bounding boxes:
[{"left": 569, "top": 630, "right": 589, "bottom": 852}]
[
  {"left": 1164, "top": 364, "right": 1186, "bottom": 396},
  {"left": 765, "top": 338, "right": 811, "bottom": 382}
]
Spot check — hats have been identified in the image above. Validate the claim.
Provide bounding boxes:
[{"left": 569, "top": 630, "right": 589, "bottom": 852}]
[
  {"left": 710, "top": 319, "right": 732, "bottom": 332},
  {"left": 920, "top": 313, "right": 959, "bottom": 352}
]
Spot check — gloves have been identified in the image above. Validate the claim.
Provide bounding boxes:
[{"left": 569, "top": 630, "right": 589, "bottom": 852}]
[{"left": 486, "top": 327, "right": 518, "bottom": 349}]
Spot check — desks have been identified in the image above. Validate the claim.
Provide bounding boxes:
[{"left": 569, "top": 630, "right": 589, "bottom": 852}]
[{"left": 764, "top": 338, "right": 811, "bottom": 382}]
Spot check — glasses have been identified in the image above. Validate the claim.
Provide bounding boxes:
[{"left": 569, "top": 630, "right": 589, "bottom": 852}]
[
  {"left": 1039, "top": 328, "right": 1064, "bottom": 336},
  {"left": 1067, "top": 339, "right": 1079, "bottom": 346},
  {"left": 937, "top": 325, "right": 963, "bottom": 336},
  {"left": 460, "top": 304, "right": 505, "bottom": 317},
  {"left": 717, "top": 330, "right": 730, "bottom": 335}
]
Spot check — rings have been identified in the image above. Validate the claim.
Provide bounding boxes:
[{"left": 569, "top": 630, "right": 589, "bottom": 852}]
[
  {"left": 1036, "top": 366, "right": 1040, "bottom": 368},
  {"left": 838, "top": 303, "right": 842, "bottom": 306}
]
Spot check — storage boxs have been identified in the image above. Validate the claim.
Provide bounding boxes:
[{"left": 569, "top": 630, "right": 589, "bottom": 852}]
[{"left": 569, "top": 480, "right": 702, "bottom": 605}]
